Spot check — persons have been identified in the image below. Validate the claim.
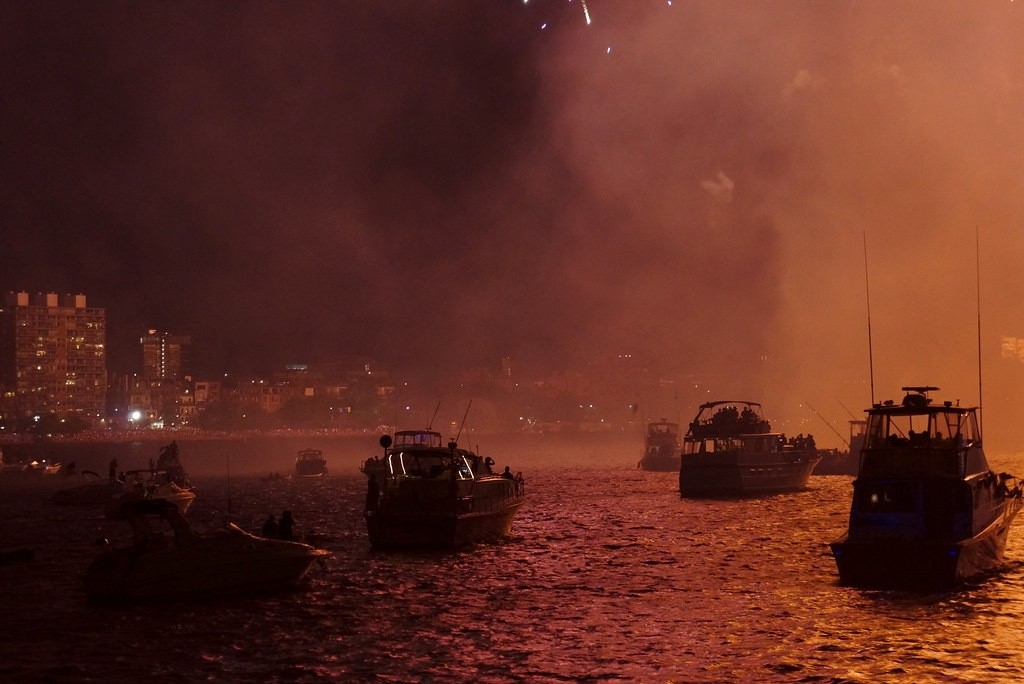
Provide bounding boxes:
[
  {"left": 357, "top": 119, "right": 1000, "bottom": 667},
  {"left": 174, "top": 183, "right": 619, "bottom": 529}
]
[
  {"left": 262, "top": 511, "right": 295, "bottom": 540},
  {"left": 717, "top": 406, "right": 752, "bottom": 421},
  {"left": 269, "top": 472, "right": 280, "bottom": 478},
  {"left": 888, "top": 430, "right": 963, "bottom": 449},
  {"left": 778, "top": 432, "right": 816, "bottom": 448},
  {"left": 479, "top": 455, "right": 514, "bottom": 480}
]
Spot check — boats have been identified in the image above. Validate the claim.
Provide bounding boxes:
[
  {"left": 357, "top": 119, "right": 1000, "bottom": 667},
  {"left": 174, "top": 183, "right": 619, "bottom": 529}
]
[
  {"left": 634, "top": 226, "right": 1024, "bottom": 587},
  {"left": 0, "top": 399, "right": 526, "bottom": 605}
]
